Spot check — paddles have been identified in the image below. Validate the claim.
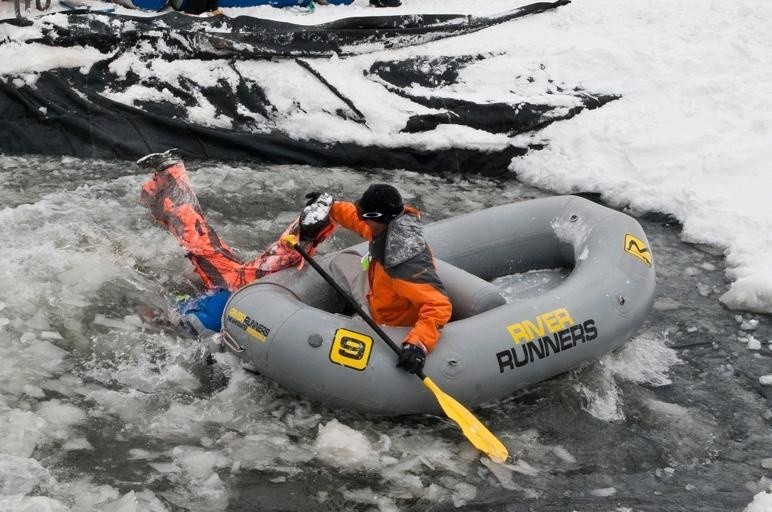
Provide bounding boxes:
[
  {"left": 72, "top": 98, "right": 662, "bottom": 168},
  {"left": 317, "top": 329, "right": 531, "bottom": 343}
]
[{"left": 283, "top": 235, "right": 508, "bottom": 463}]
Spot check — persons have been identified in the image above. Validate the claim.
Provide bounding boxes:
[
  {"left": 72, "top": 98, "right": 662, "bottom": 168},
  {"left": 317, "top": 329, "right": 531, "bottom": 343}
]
[
  {"left": 136, "top": 149, "right": 338, "bottom": 380},
  {"left": 303, "top": 183, "right": 453, "bottom": 374}
]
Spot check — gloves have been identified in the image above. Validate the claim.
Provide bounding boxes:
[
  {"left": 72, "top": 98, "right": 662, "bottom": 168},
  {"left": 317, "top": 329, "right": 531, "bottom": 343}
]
[
  {"left": 304, "top": 192, "right": 335, "bottom": 207},
  {"left": 397, "top": 342, "right": 426, "bottom": 375}
]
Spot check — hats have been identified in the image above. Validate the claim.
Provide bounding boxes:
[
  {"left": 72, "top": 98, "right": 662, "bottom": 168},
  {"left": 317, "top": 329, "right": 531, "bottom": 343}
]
[{"left": 355, "top": 184, "right": 403, "bottom": 225}]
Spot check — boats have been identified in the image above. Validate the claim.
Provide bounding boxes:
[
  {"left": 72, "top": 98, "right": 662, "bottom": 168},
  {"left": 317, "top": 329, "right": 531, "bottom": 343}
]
[{"left": 217, "top": 195, "right": 655, "bottom": 415}]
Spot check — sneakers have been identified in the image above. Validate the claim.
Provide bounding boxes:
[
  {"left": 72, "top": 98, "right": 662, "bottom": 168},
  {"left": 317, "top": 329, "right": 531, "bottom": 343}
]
[
  {"left": 136, "top": 148, "right": 184, "bottom": 172},
  {"left": 297, "top": 192, "right": 339, "bottom": 243}
]
